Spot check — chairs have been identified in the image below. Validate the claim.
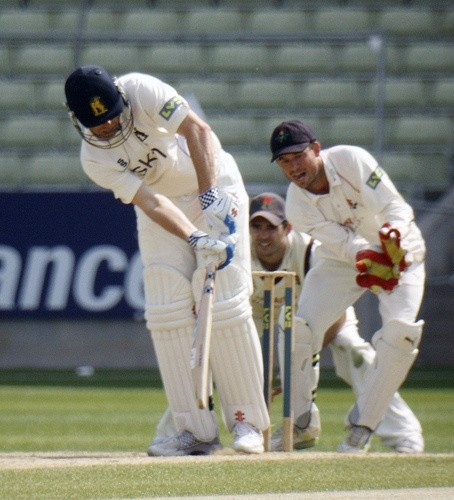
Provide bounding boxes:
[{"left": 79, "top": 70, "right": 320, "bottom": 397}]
[{"left": 0, "top": 0, "right": 454, "bottom": 203}]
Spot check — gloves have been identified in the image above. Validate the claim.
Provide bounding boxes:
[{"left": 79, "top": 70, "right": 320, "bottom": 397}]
[
  {"left": 193, "top": 189, "right": 240, "bottom": 271},
  {"left": 355, "top": 223, "right": 407, "bottom": 295}
]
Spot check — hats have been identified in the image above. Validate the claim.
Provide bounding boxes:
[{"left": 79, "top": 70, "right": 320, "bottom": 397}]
[
  {"left": 270, "top": 119, "right": 317, "bottom": 163},
  {"left": 65, "top": 65, "right": 123, "bottom": 129},
  {"left": 250, "top": 192, "right": 287, "bottom": 226}
]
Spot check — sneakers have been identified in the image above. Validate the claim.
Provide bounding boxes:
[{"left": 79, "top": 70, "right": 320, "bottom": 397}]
[
  {"left": 232, "top": 423, "right": 264, "bottom": 454},
  {"left": 270, "top": 424, "right": 317, "bottom": 451},
  {"left": 395, "top": 434, "right": 424, "bottom": 454},
  {"left": 147, "top": 425, "right": 220, "bottom": 457},
  {"left": 338, "top": 424, "right": 373, "bottom": 453}
]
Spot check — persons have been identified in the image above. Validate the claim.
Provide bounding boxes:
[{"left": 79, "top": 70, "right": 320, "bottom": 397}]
[
  {"left": 268, "top": 120, "right": 427, "bottom": 455},
  {"left": 153, "top": 195, "right": 423, "bottom": 455},
  {"left": 65, "top": 65, "right": 271, "bottom": 456}
]
